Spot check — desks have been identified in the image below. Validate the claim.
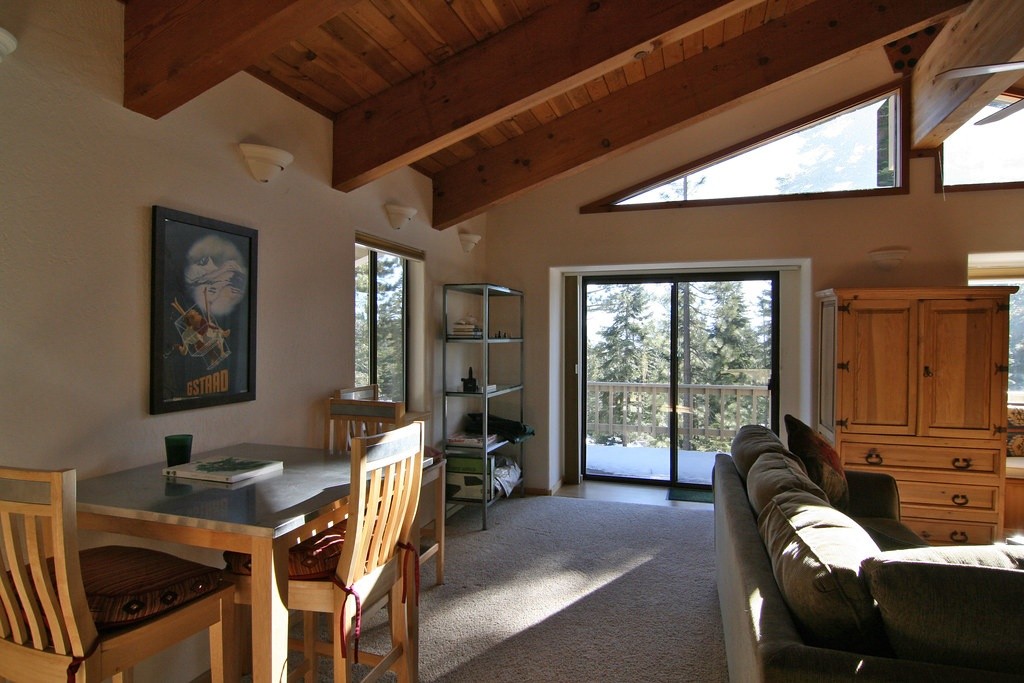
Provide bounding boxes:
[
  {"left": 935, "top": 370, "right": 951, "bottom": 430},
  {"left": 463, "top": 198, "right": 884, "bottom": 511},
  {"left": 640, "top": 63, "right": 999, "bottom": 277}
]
[{"left": 76, "top": 443, "right": 434, "bottom": 683}]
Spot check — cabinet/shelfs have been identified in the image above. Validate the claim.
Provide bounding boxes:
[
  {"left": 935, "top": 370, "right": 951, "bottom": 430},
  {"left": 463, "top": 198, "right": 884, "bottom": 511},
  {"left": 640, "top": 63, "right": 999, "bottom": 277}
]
[
  {"left": 813, "top": 286, "right": 1017, "bottom": 544},
  {"left": 441, "top": 281, "right": 526, "bottom": 528}
]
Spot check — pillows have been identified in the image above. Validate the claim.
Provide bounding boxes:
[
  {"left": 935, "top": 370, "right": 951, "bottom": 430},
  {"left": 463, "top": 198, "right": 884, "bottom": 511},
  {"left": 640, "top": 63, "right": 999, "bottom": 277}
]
[{"left": 784, "top": 413, "right": 850, "bottom": 513}]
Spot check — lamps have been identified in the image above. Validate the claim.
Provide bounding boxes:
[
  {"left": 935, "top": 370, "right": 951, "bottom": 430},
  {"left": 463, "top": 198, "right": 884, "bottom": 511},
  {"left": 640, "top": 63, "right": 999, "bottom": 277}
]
[
  {"left": 384, "top": 203, "right": 418, "bottom": 230},
  {"left": 239, "top": 142, "right": 295, "bottom": 184},
  {"left": 0, "top": 26, "right": 17, "bottom": 60},
  {"left": 459, "top": 232, "right": 481, "bottom": 254},
  {"left": 870, "top": 249, "right": 909, "bottom": 278}
]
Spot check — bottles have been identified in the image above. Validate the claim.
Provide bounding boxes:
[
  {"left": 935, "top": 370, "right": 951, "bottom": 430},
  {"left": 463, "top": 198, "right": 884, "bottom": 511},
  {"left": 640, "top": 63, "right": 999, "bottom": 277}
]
[{"left": 463, "top": 367, "right": 476, "bottom": 392}]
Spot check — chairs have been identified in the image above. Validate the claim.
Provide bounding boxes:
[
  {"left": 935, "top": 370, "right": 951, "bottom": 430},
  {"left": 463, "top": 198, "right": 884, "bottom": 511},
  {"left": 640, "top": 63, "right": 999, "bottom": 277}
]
[
  {"left": 323, "top": 397, "right": 405, "bottom": 459},
  {"left": 0, "top": 465, "right": 234, "bottom": 683},
  {"left": 335, "top": 386, "right": 447, "bottom": 586},
  {"left": 234, "top": 420, "right": 426, "bottom": 683}
]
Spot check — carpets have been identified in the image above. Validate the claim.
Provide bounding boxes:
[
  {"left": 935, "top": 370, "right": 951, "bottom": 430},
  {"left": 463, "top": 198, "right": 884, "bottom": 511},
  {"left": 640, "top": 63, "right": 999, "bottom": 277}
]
[
  {"left": 667, "top": 486, "right": 714, "bottom": 503},
  {"left": 189, "top": 496, "right": 729, "bottom": 683}
]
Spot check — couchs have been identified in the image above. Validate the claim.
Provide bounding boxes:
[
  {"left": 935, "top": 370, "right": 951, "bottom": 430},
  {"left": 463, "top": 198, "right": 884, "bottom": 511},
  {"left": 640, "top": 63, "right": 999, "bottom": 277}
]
[{"left": 712, "top": 425, "right": 1022, "bottom": 683}]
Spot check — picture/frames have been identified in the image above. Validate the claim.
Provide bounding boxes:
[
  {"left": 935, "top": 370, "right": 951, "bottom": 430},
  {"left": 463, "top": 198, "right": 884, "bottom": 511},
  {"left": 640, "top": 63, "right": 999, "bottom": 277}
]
[{"left": 150, "top": 206, "right": 258, "bottom": 415}]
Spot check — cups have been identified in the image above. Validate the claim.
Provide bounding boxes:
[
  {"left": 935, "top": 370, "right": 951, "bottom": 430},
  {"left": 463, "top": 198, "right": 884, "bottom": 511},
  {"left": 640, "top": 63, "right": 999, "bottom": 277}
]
[{"left": 164, "top": 434, "right": 193, "bottom": 467}]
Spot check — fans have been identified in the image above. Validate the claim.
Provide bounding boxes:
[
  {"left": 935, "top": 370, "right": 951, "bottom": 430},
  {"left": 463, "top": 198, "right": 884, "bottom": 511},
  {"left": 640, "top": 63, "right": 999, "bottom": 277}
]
[{"left": 934, "top": 61, "right": 1024, "bottom": 125}]
